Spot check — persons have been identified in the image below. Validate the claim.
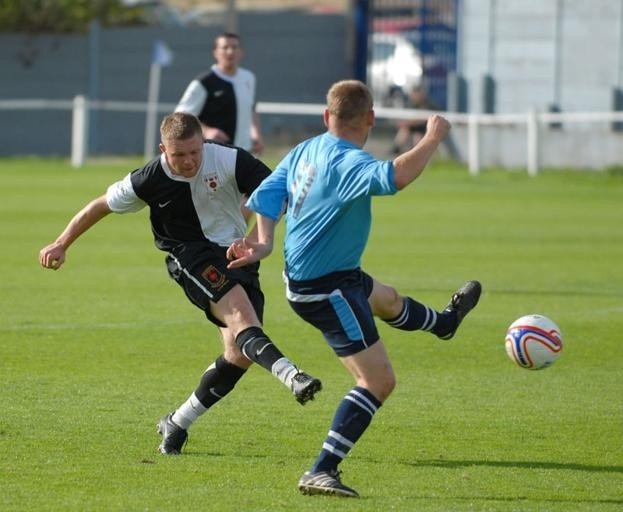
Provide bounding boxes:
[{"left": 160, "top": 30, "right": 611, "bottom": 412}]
[
  {"left": 386, "top": 86, "right": 410, "bottom": 107},
  {"left": 390, "top": 85, "right": 439, "bottom": 157},
  {"left": 226, "top": 79, "right": 482, "bottom": 494},
  {"left": 175, "top": 32, "right": 265, "bottom": 222},
  {"left": 37, "top": 111, "right": 322, "bottom": 455}
]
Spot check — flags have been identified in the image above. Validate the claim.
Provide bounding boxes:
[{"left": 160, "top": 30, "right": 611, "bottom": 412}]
[{"left": 152, "top": 42, "right": 182, "bottom": 72}]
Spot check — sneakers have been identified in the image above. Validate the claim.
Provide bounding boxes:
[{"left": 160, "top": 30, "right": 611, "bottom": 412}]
[
  {"left": 299, "top": 471, "right": 359, "bottom": 498},
  {"left": 438, "top": 281, "right": 481, "bottom": 340},
  {"left": 291, "top": 371, "right": 321, "bottom": 406},
  {"left": 156, "top": 413, "right": 188, "bottom": 455}
]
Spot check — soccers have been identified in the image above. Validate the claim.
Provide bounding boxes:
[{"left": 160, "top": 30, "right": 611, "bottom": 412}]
[{"left": 505, "top": 314, "right": 563, "bottom": 368}]
